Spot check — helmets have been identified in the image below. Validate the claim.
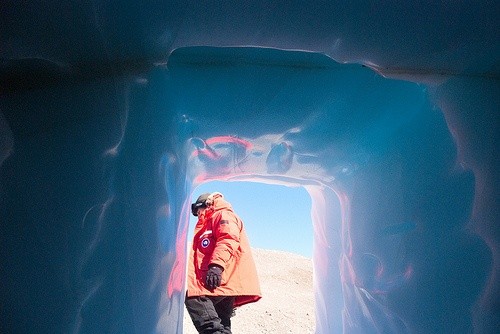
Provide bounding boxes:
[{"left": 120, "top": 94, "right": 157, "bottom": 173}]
[{"left": 192, "top": 193, "right": 211, "bottom": 216}]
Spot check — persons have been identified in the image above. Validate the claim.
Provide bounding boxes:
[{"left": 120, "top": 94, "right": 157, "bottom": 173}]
[{"left": 184, "top": 193, "right": 263, "bottom": 334}]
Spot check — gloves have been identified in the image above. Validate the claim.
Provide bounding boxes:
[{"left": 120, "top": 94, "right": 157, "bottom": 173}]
[{"left": 205, "top": 266, "right": 223, "bottom": 290}]
